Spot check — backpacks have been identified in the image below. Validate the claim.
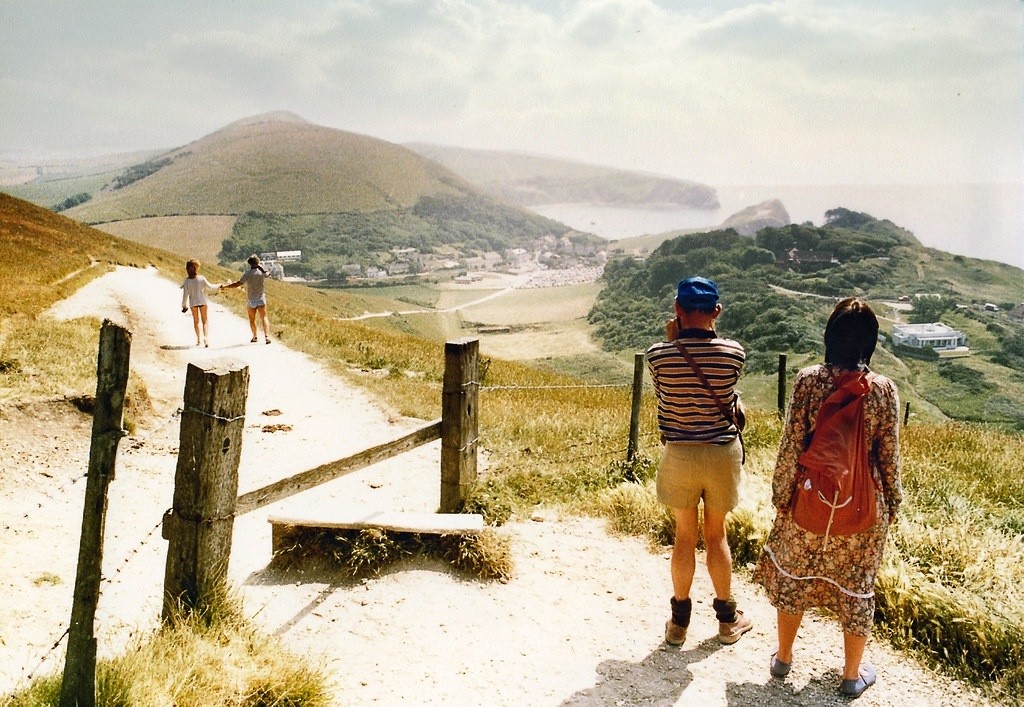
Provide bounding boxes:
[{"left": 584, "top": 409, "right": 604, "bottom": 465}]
[{"left": 791, "top": 363, "right": 876, "bottom": 535}]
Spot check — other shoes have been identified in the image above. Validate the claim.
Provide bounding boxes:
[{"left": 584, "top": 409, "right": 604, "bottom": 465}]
[
  {"left": 204, "top": 341, "right": 208, "bottom": 347},
  {"left": 251, "top": 337, "right": 258, "bottom": 342},
  {"left": 769, "top": 644, "right": 792, "bottom": 678},
  {"left": 195, "top": 342, "right": 200, "bottom": 347},
  {"left": 265, "top": 335, "right": 272, "bottom": 344},
  {"left": 842, "top": 665, "right": 876, "bottom": 697}
]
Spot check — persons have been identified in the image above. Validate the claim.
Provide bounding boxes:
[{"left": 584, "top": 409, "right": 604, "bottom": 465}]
[
  {"left": 220, "top": 254, "right": 272, "bottom": 344},
  {"left": 746, "top": 297, "right": 903, "bottom": 697},
  {"left": 181, "top": 260, "right": 222, "bottom": 348},
  {"left": 645, "top": 276, "right": 755, "bottom": 647}
]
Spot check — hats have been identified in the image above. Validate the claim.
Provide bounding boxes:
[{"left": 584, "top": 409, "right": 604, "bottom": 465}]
[{"left": 677, "top": 277, "right": 719, "bottom": 309}]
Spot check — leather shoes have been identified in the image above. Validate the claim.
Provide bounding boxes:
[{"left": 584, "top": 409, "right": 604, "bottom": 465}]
[
  {"left": 666, "top": 615, "right": 687, "bottom": 645},
  {"left": 718, "top": 608, "right": 754, "bottom": 643}
]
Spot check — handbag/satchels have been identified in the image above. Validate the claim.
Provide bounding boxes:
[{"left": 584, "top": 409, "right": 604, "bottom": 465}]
[{"left": 733, "top": 391, "right": 746, "bottom": 433}]
